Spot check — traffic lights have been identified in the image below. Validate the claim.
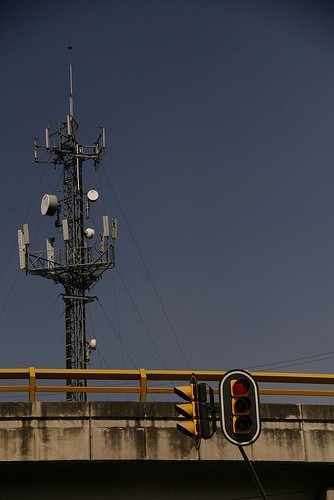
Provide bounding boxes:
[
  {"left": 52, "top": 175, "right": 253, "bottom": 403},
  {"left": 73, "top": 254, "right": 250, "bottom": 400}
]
[
  {"left": 219, "top": 368, "right": 262, "bottom": 446},
  {"left": 172, "top": 379, "right": 202, "bottom": 440}
]
